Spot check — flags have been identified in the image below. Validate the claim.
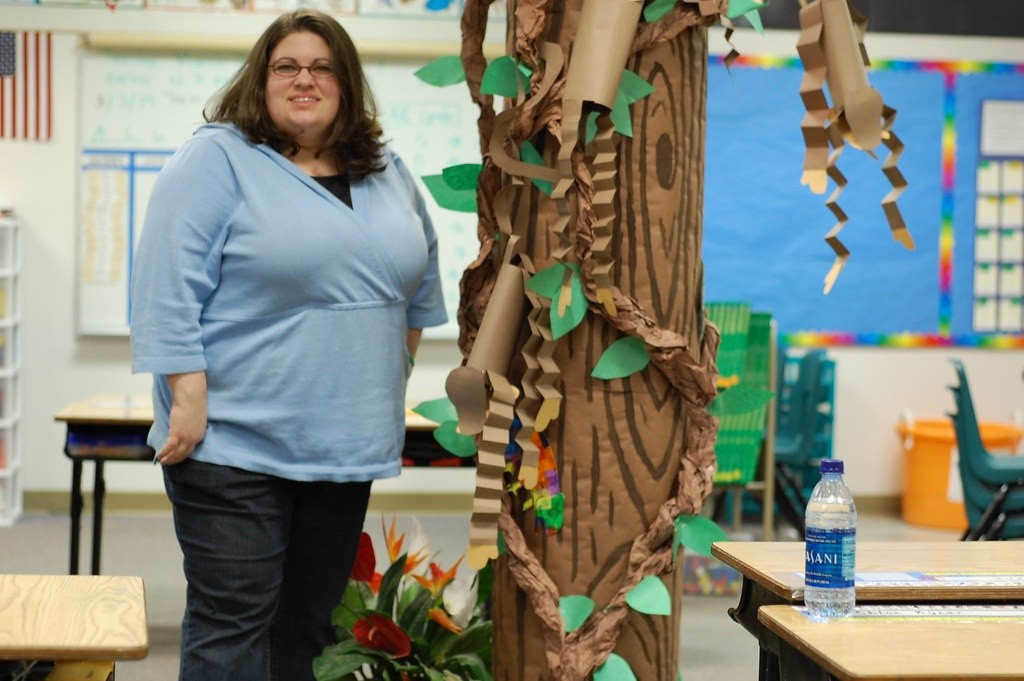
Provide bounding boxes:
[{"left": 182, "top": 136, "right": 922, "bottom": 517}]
[{"left": 1, "top": 28, "right": 54, "bottom": 143}]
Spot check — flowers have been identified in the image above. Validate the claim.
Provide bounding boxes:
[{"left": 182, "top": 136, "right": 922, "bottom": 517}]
[{"left": 311, "top": 511, "right": 494, "bottom": 681}]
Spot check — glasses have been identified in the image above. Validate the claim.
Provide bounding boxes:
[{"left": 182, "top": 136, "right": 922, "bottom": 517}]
[{"left": 267, "top": 61, "right": 339, "bottom": 78}]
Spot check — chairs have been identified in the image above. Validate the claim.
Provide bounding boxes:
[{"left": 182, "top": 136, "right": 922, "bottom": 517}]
[
  {"left": 942, "top": 358, "right": 1024, "bottom": 541},
  {"left": 716, "top": 336, "right": 836, "bottom": 524}
]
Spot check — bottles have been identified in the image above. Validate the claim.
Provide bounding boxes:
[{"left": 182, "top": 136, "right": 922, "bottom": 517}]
[{"left": 804, "top": 459, "right": 857, "bottom": 618}]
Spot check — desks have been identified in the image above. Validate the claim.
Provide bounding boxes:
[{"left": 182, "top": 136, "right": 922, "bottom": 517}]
[
  {"left": 710, "top": 541, "right": 1024, "bottom": 640},
  {"left": 0, "top": 574, "right": 149, "bottom": 681},
  {"left": 757, "top": 603, "right": 1024, "bottom": 681},
  {"left": 54, "top": 392, "right": 479, "bottom": 577}
]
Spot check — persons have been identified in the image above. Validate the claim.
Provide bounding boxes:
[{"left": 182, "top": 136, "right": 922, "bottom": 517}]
[{"left": 127, "top": 7, "right": 450, "bottom": 680}]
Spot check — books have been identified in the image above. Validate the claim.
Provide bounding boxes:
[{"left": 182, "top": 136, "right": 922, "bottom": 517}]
[{"left": 701, "top": 303, "right": 773, "bottom": 486}]
